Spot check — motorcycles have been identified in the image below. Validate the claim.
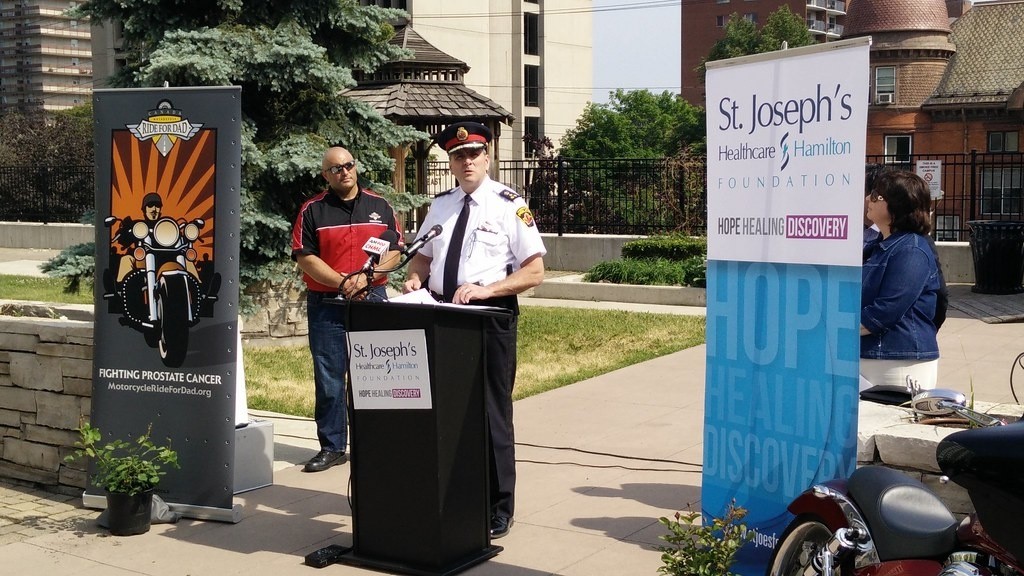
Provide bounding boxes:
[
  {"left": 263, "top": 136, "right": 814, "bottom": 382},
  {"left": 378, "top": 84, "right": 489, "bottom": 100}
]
[{"left": 768, "top": 389, "right": 1024, "bottom": 576}]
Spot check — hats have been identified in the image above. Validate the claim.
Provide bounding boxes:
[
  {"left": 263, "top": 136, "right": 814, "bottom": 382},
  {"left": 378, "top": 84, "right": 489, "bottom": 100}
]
[{"left": 437, "top": 123, "right": 493, "bottom": 154}]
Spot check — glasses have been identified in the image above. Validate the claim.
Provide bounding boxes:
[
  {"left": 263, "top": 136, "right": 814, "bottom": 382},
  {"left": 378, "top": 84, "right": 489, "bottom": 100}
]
[
  {"left": 870, "top": 190, "right": 885, "bottom": 202},
  {"left": 324, "top": 160, "right": 358, "bottom": 175}
]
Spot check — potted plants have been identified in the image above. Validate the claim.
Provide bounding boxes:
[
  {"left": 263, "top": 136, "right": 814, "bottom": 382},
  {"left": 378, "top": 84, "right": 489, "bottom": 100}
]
[{"left": 64, "top": 413, "right": 181, "bottom": 537}]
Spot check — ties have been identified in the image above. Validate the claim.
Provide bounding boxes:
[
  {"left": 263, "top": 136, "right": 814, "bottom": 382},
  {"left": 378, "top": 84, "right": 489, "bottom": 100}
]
[{"left": 442, "top": 195, "right": 470, "bottom": 304}]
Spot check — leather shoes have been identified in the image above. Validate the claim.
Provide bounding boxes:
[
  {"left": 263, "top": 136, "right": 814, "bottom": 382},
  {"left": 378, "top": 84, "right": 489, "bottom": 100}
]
[
  {"left": 303, "top": 448, "right": 348, "bottom": 472},
  {"left": 489, "top": 515, "right": 515, "bottom": 539}
]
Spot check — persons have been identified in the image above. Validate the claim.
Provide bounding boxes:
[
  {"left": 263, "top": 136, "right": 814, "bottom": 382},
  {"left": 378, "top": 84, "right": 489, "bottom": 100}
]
[
  {"left": 291, "top": 147, "right": 405, "bottom": 472},
  {"left": 860, "top": 161, "right": 948, "bottom": 391},
  {"left": 403, "top": 122, "right": 547, "bottom": 538}
]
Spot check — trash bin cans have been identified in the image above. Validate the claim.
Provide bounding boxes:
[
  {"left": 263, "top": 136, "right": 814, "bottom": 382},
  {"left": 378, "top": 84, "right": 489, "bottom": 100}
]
[{"left": 965, "top": 219, "right": 1024, "bottom": 296}]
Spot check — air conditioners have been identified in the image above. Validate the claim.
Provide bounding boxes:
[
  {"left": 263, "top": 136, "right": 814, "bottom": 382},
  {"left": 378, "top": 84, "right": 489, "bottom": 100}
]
[{"left": 879, "top": 93, "right": 892, "bottom": 103}]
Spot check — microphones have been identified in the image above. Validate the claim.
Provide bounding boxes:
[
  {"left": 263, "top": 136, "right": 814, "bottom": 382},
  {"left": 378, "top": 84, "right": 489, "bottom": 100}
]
[
  {"left": 359, "top": 230, "right": 397, "bottom": 273},
  {"left": 405, "top": 224, "right": 443, "bottom": 256}
]
